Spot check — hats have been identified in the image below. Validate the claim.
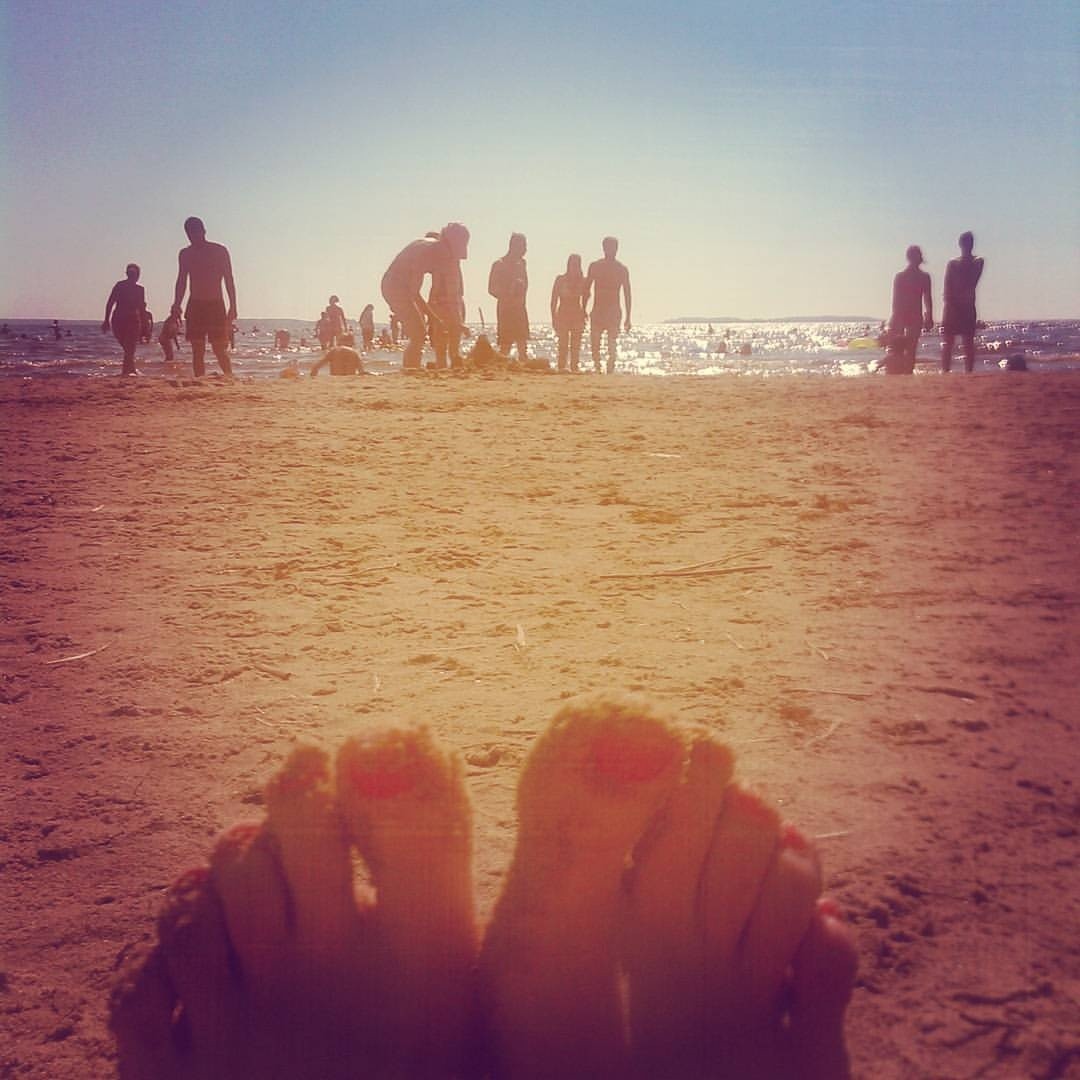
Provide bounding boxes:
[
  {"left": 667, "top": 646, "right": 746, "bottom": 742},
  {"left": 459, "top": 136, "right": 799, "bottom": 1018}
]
[{"left": 445, "top": 223, "right": 468, "bottom": 258}]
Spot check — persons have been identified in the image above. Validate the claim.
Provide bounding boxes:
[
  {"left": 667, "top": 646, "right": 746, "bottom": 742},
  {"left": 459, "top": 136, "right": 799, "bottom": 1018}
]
[
  {"left": 109, "top": 690, "right": 861, "bottom": 1080},
  {"left": 0, "top": 217, "right": 631, "bottom": 377},
  {"left": 717, "top": 230, "right": 1027, "bottom": 374}
]
